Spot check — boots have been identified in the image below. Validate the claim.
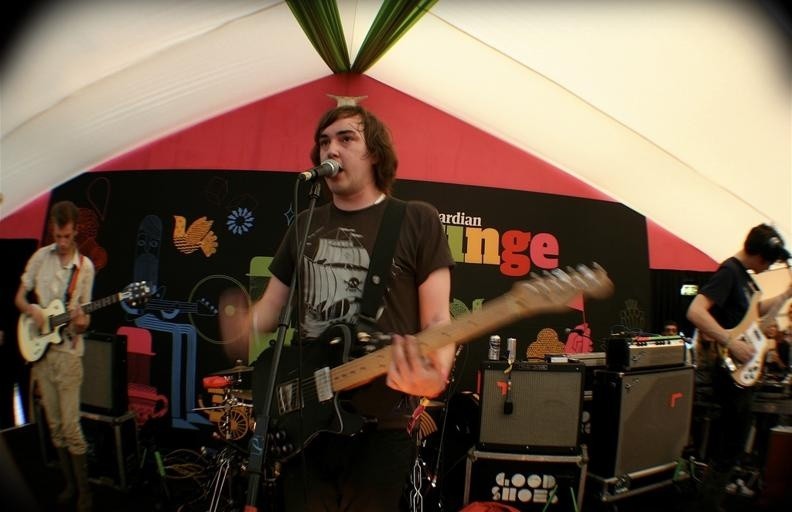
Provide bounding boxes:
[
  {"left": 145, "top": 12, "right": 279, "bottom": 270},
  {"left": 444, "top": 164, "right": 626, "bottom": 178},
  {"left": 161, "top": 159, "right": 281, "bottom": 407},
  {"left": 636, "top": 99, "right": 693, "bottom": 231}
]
[{"left": 56, "top": 448, "right": 93, "bottom": 512}]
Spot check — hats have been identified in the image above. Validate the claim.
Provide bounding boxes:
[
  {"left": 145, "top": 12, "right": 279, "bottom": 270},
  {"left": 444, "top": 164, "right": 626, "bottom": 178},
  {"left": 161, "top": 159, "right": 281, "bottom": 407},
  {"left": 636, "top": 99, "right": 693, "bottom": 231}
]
[{"left": 745, "top": 224, "right": 789, "bottom": 260}]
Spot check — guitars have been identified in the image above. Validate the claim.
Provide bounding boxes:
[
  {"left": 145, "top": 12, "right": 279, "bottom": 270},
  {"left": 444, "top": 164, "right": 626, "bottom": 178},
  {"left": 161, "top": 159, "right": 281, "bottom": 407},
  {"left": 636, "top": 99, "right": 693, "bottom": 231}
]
[
  {"left": 711, "top": 287, "right": 792, "bottom": 390},
  {"left": 17, "top": 281, "right": 150, "bottom": 364},
  {"left": 250, "top": 261, "right": 614, "bottom": 468}
]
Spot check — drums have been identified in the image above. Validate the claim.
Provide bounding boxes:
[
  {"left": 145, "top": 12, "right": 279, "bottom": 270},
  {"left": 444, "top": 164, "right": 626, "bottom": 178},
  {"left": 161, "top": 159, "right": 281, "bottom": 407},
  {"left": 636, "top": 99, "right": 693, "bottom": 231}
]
[{"left": 224, "top": 389, "right": 253, "bottom": 419}]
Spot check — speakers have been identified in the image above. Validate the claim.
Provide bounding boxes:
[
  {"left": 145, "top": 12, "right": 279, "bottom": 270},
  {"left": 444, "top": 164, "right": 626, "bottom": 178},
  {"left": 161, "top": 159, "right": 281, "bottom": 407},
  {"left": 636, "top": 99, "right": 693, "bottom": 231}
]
[
  {"left": 587, "top": 363, "right": 696, "bottom": 479},
  {"left": 474, "top": 360, "right": 588, "bottom": 456},
  {"left": 78, "top": 334, "right": 130, "bottom": 418}
]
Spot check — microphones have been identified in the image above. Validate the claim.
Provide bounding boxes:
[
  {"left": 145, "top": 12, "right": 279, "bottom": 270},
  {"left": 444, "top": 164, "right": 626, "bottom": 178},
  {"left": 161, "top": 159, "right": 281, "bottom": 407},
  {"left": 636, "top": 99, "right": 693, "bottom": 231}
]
[
  {"left": 503, "top": 378, "right": 513, "bottom": 415},
  {"left": 298, "top": 159, "right": 340, "bottom": 183}
]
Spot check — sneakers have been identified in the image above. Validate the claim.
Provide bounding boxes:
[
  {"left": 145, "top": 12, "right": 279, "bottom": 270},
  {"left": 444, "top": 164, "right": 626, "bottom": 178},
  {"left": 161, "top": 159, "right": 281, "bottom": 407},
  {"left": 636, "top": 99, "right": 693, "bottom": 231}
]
[{"left": 723, "top": 478, "right": 753, "bottom": 495}]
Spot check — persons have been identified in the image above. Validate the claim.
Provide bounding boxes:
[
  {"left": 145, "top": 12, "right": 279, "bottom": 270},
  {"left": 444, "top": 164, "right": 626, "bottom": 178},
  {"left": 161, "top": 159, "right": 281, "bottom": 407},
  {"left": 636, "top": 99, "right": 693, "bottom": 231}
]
[
  {"left": 15, "top": 200, "right": 96, "bottom": 511},
  {"left": 760, "top": 316, "right": 792, "bottom": 382},
  {"left": 659, "top": 320, "right": 680, "bottom": 337},
  {"left": 215, "top": 104, "right": 455, "bottom": 512},
  {"left": 685, "top": 222, "right": 790, "bottom": 498}
]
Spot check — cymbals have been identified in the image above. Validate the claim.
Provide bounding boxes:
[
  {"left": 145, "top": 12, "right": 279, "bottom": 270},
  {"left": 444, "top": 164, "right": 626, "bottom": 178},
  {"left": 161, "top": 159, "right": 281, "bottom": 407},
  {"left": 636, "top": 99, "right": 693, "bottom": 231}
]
[{"left": 209, "top": 364, "right": 255, "bottom": 376}]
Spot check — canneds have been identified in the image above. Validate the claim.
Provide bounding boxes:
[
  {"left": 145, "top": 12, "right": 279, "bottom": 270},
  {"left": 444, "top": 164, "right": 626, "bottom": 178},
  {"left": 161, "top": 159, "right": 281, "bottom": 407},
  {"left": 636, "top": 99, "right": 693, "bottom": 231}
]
[
  {"left": 487, "top": 334, "right": 501, "bottom": 361},
  {"left": 507, "top": 338, "right": 517, "bottom": 360}
]
